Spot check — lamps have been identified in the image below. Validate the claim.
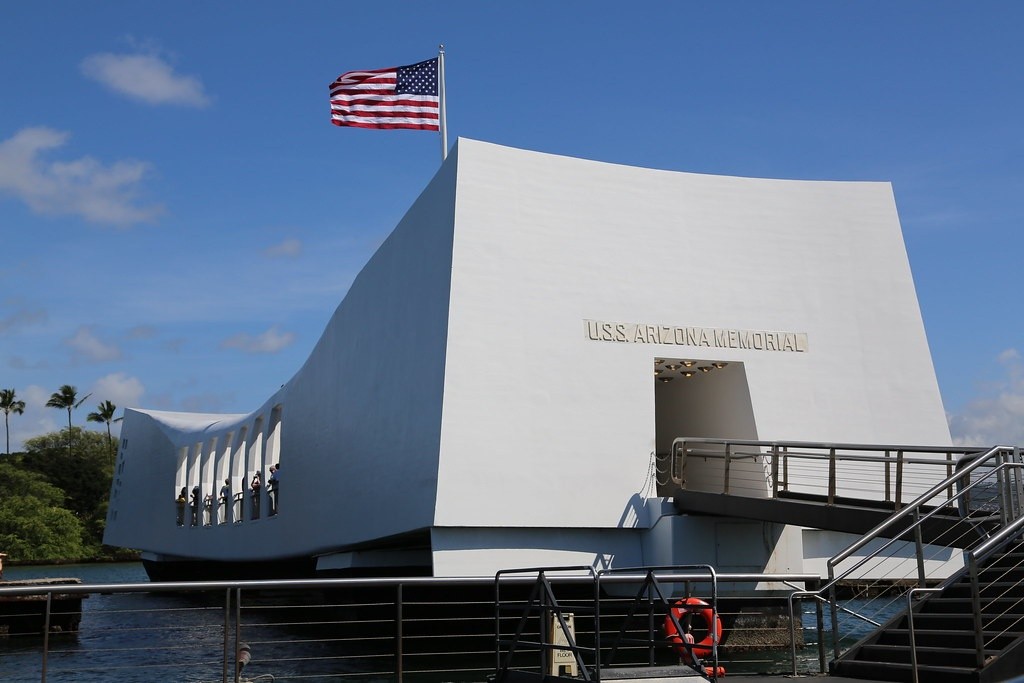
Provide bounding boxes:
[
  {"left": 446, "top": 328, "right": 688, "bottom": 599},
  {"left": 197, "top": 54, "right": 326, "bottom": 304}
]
[
  {"left": 654, "top": 360, "right": 665, "bottom": 366},
  {"left": 654, "top": 370, "right": 662, "bottom": 376},
  {"left": 680, "top": 371, "right": 696, "bottom": 377},
  {"left": 665, "top": 364, "right": 682, "bottom": 371},
  {"left": 658, "top": 377, "right": 674, "bottom": 383},
  {"left": 712, "top": 362, "right": 727, "bottom": 369},
  {"left": 698, "top": 367, "right": 713, "bottom": 373},
  {"left": 679, "top": 360, "right": 697, "bottom": 367}
]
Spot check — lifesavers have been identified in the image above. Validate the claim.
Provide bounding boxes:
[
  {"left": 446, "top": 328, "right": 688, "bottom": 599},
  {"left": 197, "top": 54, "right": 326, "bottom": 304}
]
[{"left": 664, "top": 598, "right": 722, "bottom": 657}]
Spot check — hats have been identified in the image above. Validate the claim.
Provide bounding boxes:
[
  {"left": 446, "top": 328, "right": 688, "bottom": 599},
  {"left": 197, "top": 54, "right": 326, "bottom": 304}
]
[{"left": 255, "top": 470, "right": 261, "bottom": 475}]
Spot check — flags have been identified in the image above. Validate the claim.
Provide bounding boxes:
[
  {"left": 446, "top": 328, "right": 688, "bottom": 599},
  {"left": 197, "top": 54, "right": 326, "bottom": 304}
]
[{"left": 329, "top": 56, "right": 441, "bottom": 133}]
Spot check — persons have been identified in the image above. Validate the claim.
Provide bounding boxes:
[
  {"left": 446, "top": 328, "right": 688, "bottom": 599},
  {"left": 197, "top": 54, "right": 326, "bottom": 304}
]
[
  {"left": 178, "top": 487, "right": 186, "bottom": 526},
  {"left": 220, "top": 479, "right": 229, "bottom": 523},
  {"left": 0, "top": 552, "right": 8, "bottom": 581},
  {"left": 189, "top": 486, "right": 198, "bottom": 526},
  {"left": 251, "top": 463, "right": 280, "bottom": 518}
]
